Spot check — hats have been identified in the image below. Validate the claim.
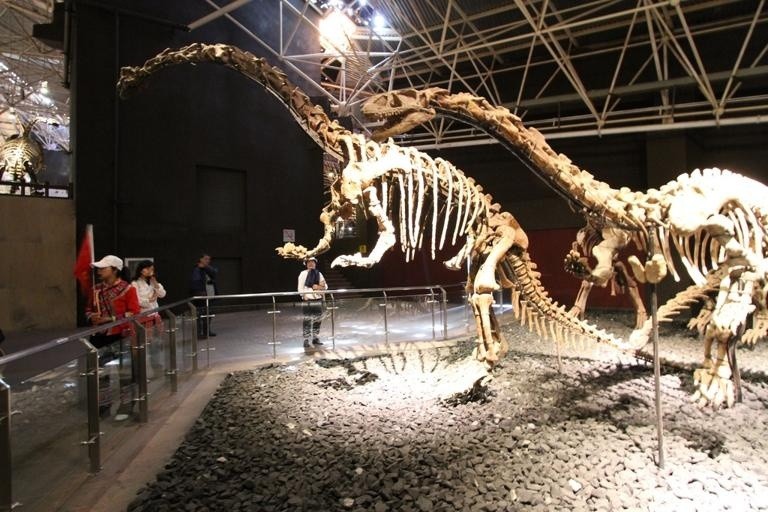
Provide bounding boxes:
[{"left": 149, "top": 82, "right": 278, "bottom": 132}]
[
  {"left": 89, "top": 255, "right": 124, "bottom": 272},
  {"left": 303, "top": 257, "right": 318, "bottom": 265}
]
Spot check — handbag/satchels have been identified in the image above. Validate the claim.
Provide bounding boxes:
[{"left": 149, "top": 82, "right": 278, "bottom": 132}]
[{"left": 89, "top": 333, "right": 122, "bottom": 367}]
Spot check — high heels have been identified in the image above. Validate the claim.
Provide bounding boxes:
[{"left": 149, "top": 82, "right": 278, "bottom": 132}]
[
  {"left": 117, "top": 401, "right": 136, "bottom": 414},
  {"left": 99, "top": 407, "right": 110, "bottom": 417}
]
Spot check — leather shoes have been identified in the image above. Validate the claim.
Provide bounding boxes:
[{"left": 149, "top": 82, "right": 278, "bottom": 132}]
[
  {"left": 304, "top": 338, "right": 323, "bottom": 346},
  {"left": 197, "top": 331, "right": 216, "bottom": 338}
]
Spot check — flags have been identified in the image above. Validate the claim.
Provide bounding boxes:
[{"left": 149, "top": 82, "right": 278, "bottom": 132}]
[{"left": 72, "top": 226, "right": 94, "bottom": 305}]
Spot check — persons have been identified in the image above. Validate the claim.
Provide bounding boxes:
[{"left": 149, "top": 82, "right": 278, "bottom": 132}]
[
  {"left": 191, "top": 254, "right": 218, "bottom": 340},
  {"left": 85, "top": 256, "right": 166, "bottom": 420},
  {"left": 298, "top": 258, "right": 329, "bottom": 348}
]
